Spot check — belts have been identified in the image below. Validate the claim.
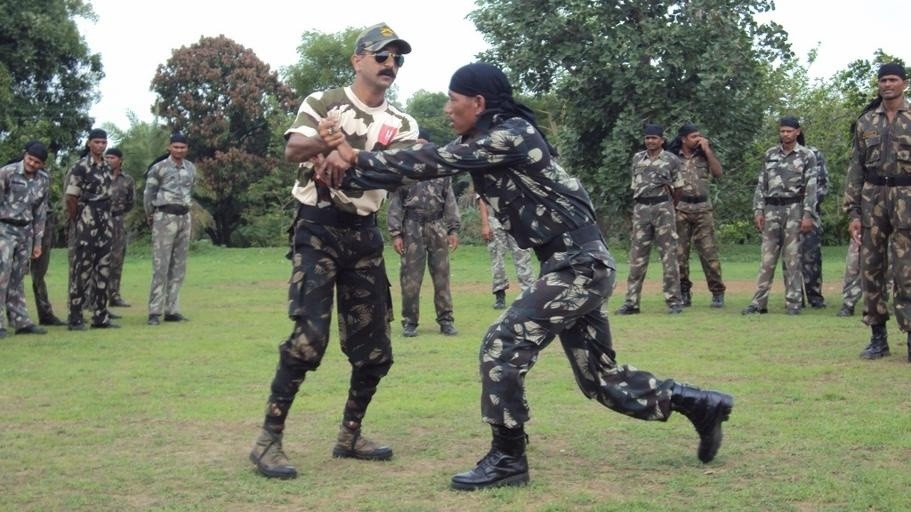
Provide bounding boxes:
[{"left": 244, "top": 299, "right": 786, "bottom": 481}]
[
  {"left": 636, "top": 192, "right": 669, "bottom": 205},
  {"left": 85, "top": 199, "right": 125, "bottom": 218},
  {"left": 158, "top": 205, "right": 190, "bottom": 216},
  {"left": 1, "top": 216, "right": 33, "bottom": 226},
  {"left": 867, "top": 176, "right": 911, "bottom": 187},
  {"left": 680, "top": 194, "right": 708, "bottom": 203},
  {"left": 297, "top": 204, "right": 379, "bottom": 230},
  {"left": 534, "top": 224, "right": 604, "bottom": 262},
  {"left": 766, "top": 196, "right": 801, "bottom": 206},
  {"left": 406, "top": 207, "right": 443, "bottom": 224}
]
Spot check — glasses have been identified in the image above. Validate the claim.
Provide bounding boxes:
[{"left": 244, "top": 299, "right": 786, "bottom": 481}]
[{"left": 361, "top": 49, "right": 404, "bottom": 66}]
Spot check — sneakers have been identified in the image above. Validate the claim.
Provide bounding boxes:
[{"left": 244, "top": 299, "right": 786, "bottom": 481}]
[
  {"left": 615, "top": 305, "right": 640, "bottom": 315},
  {"left": 441, "top": 324, "right": 457, "bottom": 335},
  {"left": 741, "top": 300, "right": 854, "bottom": 316},
  {"left": 404, "top": 324, "right": 416, "bottom": 336},
  {"left": 17, "top": 298, "right": 187, "bottom": 334},
  {"left": 682, "top": 290, "right": 691, "bottom": 306},
  {"left": 712, "top": 296, "right": 724, "bottom": 307},
  {"left": 670, "top": 306, "right": 680, "bottom": 313}
]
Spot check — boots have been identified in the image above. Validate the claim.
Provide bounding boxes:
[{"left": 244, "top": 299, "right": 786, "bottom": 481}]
[
  {"left": 333, "top": 417, "right": 392, "bottom": 460},
  {"left": 670, "top": 383, "right": 732, "bottom": 462},
  {"left": 250, "top": 422, "right": 297, "bottom": 478},
  {"left": 451, "top": 425, "right": 529, "bottom": 490},
  {"left": 494, "top": 290, "right": 504, "bottom": 308},
  {"left": 860, "top": 323, "right": 889, "bottom": 359}
]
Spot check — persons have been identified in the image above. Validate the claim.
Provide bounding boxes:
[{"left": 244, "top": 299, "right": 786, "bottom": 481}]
[
  {"left": 0, "top": 129, "right": 135, "bottom": 338},
  {"left": 247, "top": 23, "right": 419, "bottom": 477},
  {"left": 838, "top": 215, "right": 896, "bottom": 318},
  {"left": 777, "top": 127, "right": 829, "bottom": 310},
  {"left": 144, "top": 136, "right": 197, "bottom": 325},
  {"left": 476, "top": 188, "right": 536, "bottom": 309},
  {"left": 842, "top": 63, "right": 911, "bottom": 363},
  {"left": 315, "top": 62, "right": 733, "bottom": 490},
  {"left": 387, "top": 131, "right": 460, "bottom": 336},
  {"left": 666, "top": 125, "right": 728, "bottom": 307},
  {"left": 613, "top": 125, "right": 684, "bottom": 314},
  {"left": 741, "top": 118, "right": 818, "bottom": 316}
]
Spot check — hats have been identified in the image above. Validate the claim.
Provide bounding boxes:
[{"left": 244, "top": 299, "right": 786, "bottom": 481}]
[{"left": 354, "top": 22, "right": 410, "bottom": 56}]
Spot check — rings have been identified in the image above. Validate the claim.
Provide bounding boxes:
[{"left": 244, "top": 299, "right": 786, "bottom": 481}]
[
  {"left": 325, "top": 167, "right": 330, "bottom": 175},
  {"left": 328, "top": 128, "right": 333, "bottom": 135}
]
